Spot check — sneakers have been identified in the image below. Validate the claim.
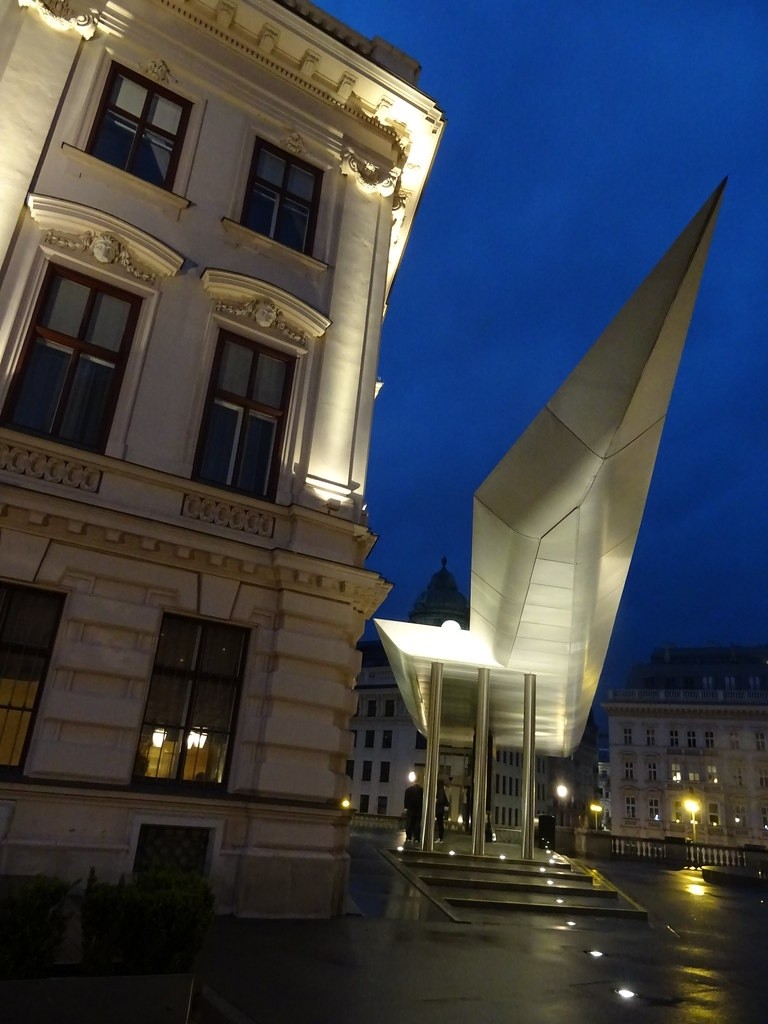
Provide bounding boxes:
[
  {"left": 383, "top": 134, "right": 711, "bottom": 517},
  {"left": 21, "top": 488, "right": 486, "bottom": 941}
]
[{"left": 434, "top": 838, "right": 444, "bottom": 844}]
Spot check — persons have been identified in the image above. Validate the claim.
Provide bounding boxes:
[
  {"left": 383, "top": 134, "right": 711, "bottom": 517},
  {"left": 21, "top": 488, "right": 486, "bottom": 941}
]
[
  {"left": 435, "top": 782, "right": 449, "bottom": 844},
  {"left": 404, "top": 780, "right": 423, "bottom": 843}
]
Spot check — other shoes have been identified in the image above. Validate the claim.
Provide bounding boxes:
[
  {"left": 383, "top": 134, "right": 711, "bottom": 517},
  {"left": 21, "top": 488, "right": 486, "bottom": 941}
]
[
  {"left": 406, "top": 838, "right": 411, "bottom": 841},
  {"left": 414, "top": 839, "right": 418, "bottom": 842}
]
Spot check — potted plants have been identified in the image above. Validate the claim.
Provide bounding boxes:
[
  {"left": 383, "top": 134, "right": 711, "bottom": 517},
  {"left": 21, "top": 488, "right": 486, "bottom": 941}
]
[{"left": 0, "top": 873, "right": 217, "bottom": 1023}]
